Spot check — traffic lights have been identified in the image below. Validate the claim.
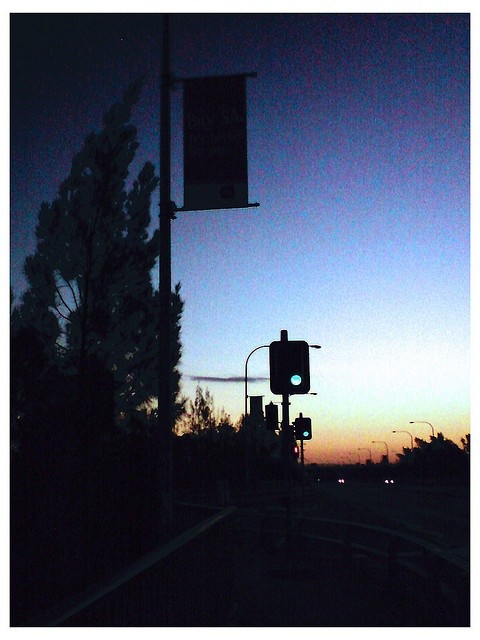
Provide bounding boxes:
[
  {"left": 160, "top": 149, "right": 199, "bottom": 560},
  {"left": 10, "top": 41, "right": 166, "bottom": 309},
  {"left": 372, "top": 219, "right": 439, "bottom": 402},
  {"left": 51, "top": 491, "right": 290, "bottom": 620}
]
[
  {"left": 296, "top": 418, "right": 312, "bottom": 439},
  {"left": 269, "top": 340, "right": 309, "bottom": 394}
]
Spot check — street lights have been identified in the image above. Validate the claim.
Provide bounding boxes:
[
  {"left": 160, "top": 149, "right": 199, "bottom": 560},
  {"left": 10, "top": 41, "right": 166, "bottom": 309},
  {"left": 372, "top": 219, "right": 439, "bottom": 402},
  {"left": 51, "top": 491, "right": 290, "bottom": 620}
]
[
  {"left": 358, "top": 448, "right": 371, "bottom": 462},
  {"left": 349, "top": 452, "right": 360, "bottom": 464},
  {"left": 340, "top": 456, "right": 350, "bottom": 465},
  {"left": 244, "top": 344, "right": 320, "bottom": 417},
  {"left": 410, "top": 422, "right": 434, "bottom": 437},
  {"left": 393, "top": 430, "right": 413, "bottom": 452},
  {"left": 372, "top": 441, "right": 389, "bottom": 463}
]
